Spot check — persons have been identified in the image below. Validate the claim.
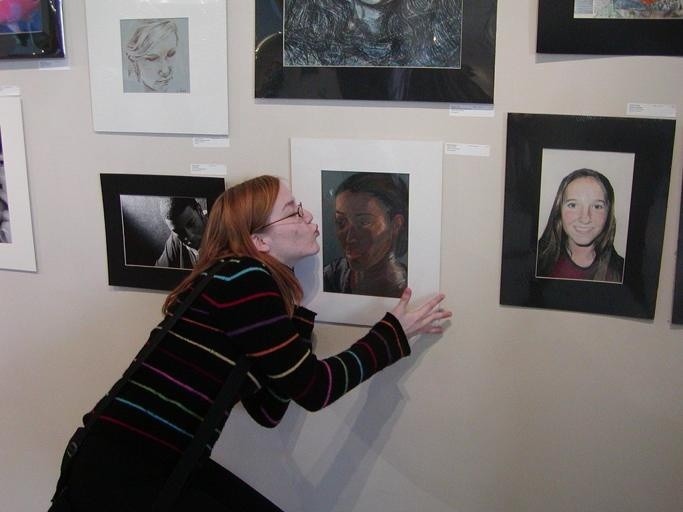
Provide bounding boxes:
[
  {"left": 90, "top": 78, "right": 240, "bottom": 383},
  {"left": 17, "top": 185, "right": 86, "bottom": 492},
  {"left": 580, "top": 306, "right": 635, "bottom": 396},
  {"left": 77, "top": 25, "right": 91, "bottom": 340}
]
[
  {"left": 125, "top": 19, "right": 179, "bottom": 93},
  {"left": 323, "top": 172, "right": 409, "bottom": 297},
  {"left": 537, "top": 168, "right": 624, "bottom": 282},
  {"left": 153, "top": 197, "right": 209, "bottom": 269},
  {"left": 48, "top": 175, "right": 453, "bottom": 512}
]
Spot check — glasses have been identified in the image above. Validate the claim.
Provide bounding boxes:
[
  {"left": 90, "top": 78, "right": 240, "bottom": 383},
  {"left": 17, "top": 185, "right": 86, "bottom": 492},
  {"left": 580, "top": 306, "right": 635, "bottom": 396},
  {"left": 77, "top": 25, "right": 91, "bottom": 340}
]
[{"left": 253, "top": 202, "right": 303, "bottom": 232}]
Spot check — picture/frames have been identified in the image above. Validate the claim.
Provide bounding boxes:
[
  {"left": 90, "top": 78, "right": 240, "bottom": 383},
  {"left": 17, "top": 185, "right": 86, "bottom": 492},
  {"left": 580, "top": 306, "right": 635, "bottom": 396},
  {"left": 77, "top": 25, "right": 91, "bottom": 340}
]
[
  {"left": 291, "top": 136, "right": 444, "bottom": 327},
  {"left": 85, "top": 1, "right": 230, "bottom": 138},
  {"left": 671, "top": 186, "right": 683, "bottom": 326},
  {"left": 499, "top": 112, "right": 677, "bottom": 321},
  {"left": 99, "top": 173, "right": 225, "bottom": 292},
  {"left": 535, "top": 0, "right": 683, "bottom": 58},
  {"left": 254, "top": 0, "right": 498, "bottom": 111},
  {"left": 1, "top": 85, "right": 38, "bottom": 273},
  {"left": 0, "top": 1, "right": 69, "bottom": 71}
]
